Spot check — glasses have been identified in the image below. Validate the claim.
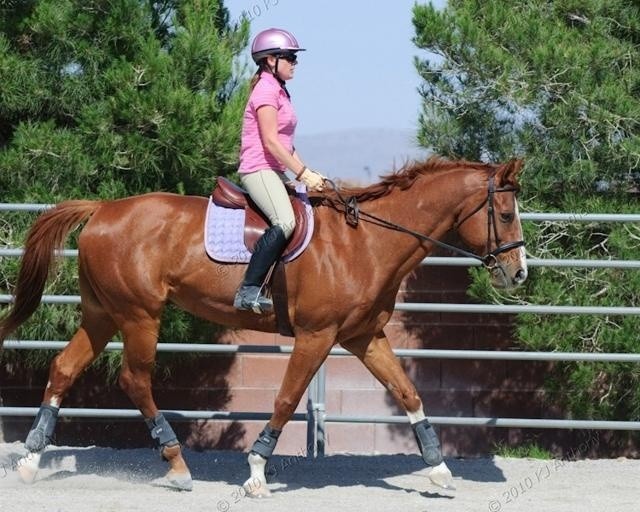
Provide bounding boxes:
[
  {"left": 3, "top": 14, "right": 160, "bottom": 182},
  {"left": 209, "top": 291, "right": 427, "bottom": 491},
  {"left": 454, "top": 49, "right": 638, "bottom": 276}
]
[{"left": 272, "top": 53, "right": 296, "bottom": 61}]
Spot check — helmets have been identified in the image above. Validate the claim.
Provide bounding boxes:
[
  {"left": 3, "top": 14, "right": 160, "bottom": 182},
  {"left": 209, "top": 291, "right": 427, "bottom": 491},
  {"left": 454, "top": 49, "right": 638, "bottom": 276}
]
[{"left": 252, "top": 28, "right": 306, "bottom": 62}]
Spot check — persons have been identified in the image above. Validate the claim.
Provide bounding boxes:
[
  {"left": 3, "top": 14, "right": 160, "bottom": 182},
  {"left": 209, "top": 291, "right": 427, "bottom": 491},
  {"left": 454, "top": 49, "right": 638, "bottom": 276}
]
[{"left": 232, "top": 28, "right": 326, "bottom": 312}]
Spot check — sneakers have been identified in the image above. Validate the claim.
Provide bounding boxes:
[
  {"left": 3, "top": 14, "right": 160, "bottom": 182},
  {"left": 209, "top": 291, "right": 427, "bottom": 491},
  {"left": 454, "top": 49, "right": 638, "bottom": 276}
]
[{"left": 234, "top": 286, "right": 273, "bottom": 310}]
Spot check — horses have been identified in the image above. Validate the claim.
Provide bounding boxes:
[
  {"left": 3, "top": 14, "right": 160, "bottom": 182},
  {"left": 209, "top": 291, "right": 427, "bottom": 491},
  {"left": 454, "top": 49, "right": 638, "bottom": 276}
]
[{"left": 1, "top": 155, "right": 531, "bottom": 501}]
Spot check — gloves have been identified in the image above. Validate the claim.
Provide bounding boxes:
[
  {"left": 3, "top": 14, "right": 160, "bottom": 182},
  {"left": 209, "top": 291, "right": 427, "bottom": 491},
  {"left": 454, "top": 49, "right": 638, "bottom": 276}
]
[{"left": 294, "top": 166, "right": 326, "bottom": 192}]
